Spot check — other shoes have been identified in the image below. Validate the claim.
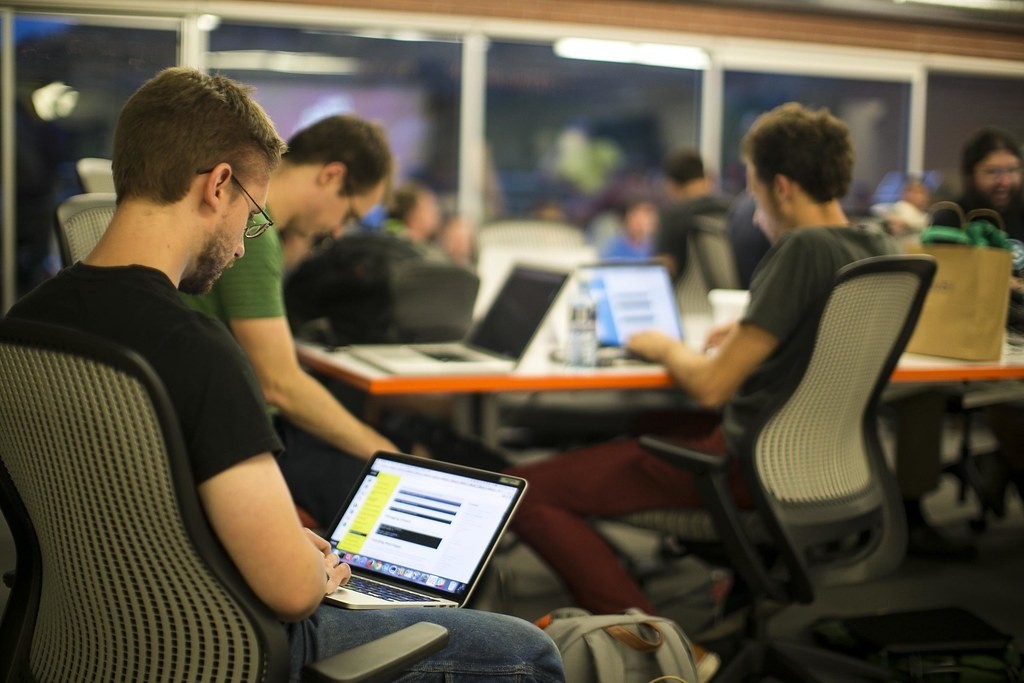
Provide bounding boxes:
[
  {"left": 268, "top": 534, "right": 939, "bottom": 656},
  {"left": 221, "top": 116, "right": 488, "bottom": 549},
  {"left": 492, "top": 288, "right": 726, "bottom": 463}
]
[{"left": 692, "top": 643, "right": 722, "bottom": 683}]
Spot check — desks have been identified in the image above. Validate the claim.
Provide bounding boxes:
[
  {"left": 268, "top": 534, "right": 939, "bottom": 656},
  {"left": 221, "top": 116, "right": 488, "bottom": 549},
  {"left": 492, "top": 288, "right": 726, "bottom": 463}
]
[{"left": 299, "top": 331, "right": 1022, "bottom": 564}]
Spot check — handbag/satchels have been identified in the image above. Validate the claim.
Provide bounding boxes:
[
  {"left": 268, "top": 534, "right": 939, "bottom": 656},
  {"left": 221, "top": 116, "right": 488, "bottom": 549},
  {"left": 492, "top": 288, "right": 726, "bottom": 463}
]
[{"left": 894, "top": 201, "right": 1013, "bottom": 363}]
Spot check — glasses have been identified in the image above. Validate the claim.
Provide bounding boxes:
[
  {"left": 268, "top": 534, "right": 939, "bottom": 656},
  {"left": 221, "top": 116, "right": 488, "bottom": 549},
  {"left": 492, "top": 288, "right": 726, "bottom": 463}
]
[{"left": 196, "top": 169, "right": 274, "bottom": 238}]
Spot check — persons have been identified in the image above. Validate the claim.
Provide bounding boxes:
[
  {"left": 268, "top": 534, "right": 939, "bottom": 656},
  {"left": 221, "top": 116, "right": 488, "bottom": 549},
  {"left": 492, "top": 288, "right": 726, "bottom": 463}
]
[
  {"left": 500, "top": 101, "right": 905, "bottom": 683},
  {"left": 176, "top": 114, "right": 511, "bottom": 567},
  {"left": 280, "top": 127, "right": 1023, "bottom": 343},
  {"left": 5, "top": 66, "right": 565, "bottom": 683}
]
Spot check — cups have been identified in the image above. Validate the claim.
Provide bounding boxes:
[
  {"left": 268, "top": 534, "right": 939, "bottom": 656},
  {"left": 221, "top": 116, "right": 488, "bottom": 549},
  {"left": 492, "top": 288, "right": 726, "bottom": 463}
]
[{"left": 706, "top": 288, "right": 750, "bottom": 327}]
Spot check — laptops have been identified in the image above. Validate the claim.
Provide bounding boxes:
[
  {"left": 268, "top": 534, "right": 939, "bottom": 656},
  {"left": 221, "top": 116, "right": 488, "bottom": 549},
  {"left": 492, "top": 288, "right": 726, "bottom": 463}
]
[
  {"left": 315, "top": 451, "right": 528, "bottom": 610},
  {"left": 348, "top": 263, "right": 572, "bottom": 375},
  {"left": 572, "top": 260, "right": 687, "bottom": 363}
]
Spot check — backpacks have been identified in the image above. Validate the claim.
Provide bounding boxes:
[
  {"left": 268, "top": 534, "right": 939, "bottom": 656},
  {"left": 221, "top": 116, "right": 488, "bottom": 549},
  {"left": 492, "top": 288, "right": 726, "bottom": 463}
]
[{"left": 531, "top": 607, "right": 697, "bottom": 683}]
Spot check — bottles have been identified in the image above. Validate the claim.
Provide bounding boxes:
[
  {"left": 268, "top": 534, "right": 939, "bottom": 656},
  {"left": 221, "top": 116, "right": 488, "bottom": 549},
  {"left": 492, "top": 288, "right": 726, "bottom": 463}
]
[{"left": 569, "top": 289, "right": 601, "bottom": 369}]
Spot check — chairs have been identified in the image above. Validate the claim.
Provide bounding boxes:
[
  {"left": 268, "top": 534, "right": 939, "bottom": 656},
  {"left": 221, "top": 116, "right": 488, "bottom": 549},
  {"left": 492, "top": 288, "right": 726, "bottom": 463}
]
[{"left": 1, "top": 203, "right": 1022, "bottom": 683}]
